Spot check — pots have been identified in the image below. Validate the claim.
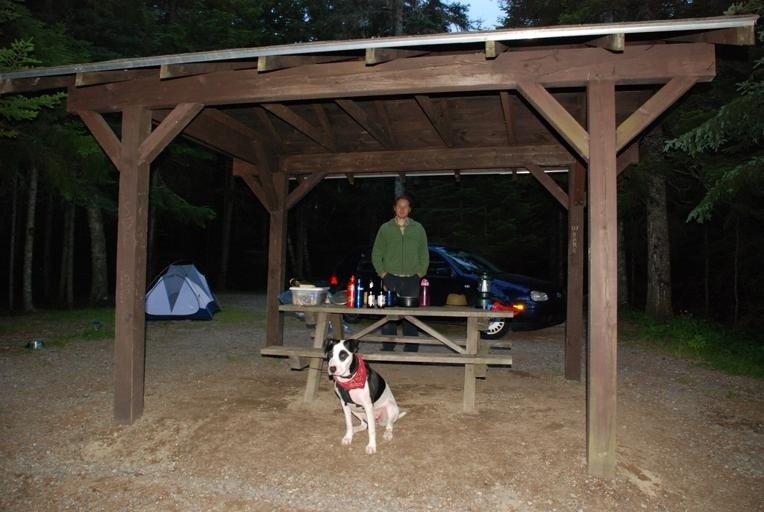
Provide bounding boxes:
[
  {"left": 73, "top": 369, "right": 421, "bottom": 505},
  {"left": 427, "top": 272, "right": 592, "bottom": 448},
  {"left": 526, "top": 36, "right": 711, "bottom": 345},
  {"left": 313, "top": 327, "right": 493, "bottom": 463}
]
[{"left": 397, "top": 295, "right": 417, "bottom": 308}]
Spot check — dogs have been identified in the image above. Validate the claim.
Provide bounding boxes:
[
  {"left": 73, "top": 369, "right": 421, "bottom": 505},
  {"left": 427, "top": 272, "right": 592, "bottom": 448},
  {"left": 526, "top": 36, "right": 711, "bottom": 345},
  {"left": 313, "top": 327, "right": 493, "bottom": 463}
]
[{"left": 323, "top": 338, "right": 408, "bottom": 455}]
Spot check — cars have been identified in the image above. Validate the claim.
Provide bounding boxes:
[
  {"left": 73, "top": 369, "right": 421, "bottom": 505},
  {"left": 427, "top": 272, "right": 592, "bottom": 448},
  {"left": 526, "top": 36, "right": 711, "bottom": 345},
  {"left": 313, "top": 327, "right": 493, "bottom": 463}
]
[{"left": 330, "top": 244, "right": 566, "bottom": 338}]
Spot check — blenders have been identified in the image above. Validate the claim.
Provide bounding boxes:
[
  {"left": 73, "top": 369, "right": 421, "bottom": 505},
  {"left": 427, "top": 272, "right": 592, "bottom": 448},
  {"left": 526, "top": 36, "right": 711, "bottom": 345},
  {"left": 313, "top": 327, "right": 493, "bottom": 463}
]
[{"left": 474, "top": 272, "right": 492, "bottom": 308}]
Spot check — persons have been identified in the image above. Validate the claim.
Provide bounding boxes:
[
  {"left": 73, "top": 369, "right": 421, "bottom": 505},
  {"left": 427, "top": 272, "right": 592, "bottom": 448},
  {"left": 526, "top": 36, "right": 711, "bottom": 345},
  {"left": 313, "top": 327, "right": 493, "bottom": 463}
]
[{"left": 371, "top": 196, "right": 432, "bottom": 353}]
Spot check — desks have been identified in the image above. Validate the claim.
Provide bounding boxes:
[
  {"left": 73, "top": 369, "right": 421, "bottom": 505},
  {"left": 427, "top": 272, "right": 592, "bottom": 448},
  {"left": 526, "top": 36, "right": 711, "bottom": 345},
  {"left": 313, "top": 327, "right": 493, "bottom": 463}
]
[{"left": 277, "top": 302, "right": 514, "bottom": 414}]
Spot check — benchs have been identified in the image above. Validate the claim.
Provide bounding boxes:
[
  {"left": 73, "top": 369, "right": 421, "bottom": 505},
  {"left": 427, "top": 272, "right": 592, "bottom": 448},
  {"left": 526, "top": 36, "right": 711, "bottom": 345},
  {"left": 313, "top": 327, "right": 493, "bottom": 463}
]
[
  {"left": 309, "top": 331, "right": 512, "bottom": 380},
  {"left": 259, "top": 344, "right": 513, "bottom": 412}
]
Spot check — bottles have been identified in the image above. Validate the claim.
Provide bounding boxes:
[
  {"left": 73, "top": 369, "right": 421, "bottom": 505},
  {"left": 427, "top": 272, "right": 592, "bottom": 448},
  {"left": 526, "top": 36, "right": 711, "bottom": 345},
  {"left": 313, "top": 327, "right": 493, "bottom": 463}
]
[
  {"left": 346, "top": 275, "right": 357, "bottom": 307},
  {"left": 355, "top": 278, "right": 363, "bottom": 308},
  {"left": 385, "top": 290, "right": 393, "bottom": 307},
  {"left": 366, "top": 280, "right": 375, "bottom": 308},
  {"left": 376, "top": 280, "right": 386, "bottom": 308},
  {"left": 418, "top": 278, "right": 430, "bottom": 308}
]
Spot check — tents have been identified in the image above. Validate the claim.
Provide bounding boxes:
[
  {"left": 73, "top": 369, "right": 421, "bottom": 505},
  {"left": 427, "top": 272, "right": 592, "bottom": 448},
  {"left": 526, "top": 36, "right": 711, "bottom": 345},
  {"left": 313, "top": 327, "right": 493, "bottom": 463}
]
[{"left": 143, "top": 260, "right": 223, "bottom": 322}]
